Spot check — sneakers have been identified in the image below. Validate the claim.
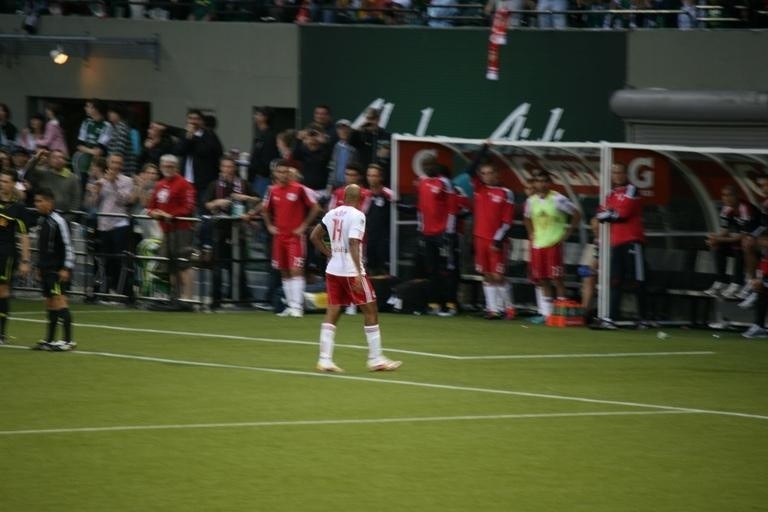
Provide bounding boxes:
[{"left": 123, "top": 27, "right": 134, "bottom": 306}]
[
  {"left": 742, "top": 324, "right": 768, "bottom": 339},
  {"left": 705, "top": 282, "right": 728, "bottom": 298},
  {"left": 277, "top": 308, "right": 290, "bottom": 316},
  {"left": 736, "top": 281, "right": 753, "bottom": 298},
  {"left": 438, "top": 304, "right": 456, "bottom": 317},
  {"left": 368, "top": 358, "right": 401, "bottom": 371},
  {"left": 720, "top": 284, "right": 743, "bottom": 298},
  {"left": 51, "top": 340, "right": 77, "bottom": 351},
  {"left": 316, "top": 362, "right": 342, "bottom": 373},
  {"left": 291, "top": 309, "right": 303, "bottom": 317},
  {"left": 738, "top": 293, "right": 758, "bottom": 309},
  {"left": 428, "top": 304, "right": 441, "bottom": 315},
  {"left": 32, "top": 339, "right": 56, "bottom": 351}
]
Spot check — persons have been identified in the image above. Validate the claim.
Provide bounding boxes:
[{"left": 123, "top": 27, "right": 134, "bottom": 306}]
[
  {"left": 0, "top": 2, "right": 768, "bottom": 37},
  {"left": 741, "top": 234, "right": 768, "bottom": 339},
  {"left": 0, "top": 97, "right": 79, "bottom": 218},
  {"left": 244, "top": 166, "right": 320, "bottom": 225},
  {"left": 705, "top": 185, "right": 761, "bottom": 298},
  {"left": 410, "top": 149, "right": 461, "bottom": 318},
  {"left": 329, "top": 163, "right": 372, "bottom": 316},
  {"left": 599, "top": 163, "right": 647, "bottom": 333},
  {"left": 248, "top": 104, "right": 402, "bottom": 184},
  {"left": 309, "top": 183, "right": 404, "bottom": 372},
  {"left": 260, "top": 162, "right": 320, "bottom": 317},
  {"left": 80, "top": 99, "right": 242, "bottom": 314},
  {"left": 366, "top": 164, "right": 395, "bottom": 255},
  {"left": 737, "top": 174, "right": 766, "bottom": 303},
  {"left": 523, "top": 169, "right": 580, "bottom": 320},
  {"left": 439, "top": 163, "right": 469, "bottom": 273},
  {"left": 32, "top": 190, "right": 79, "bottom": 350},
  {"left": 1, "top": 172, "right": 27, "bottom": 344},
  {"left": 469, "top": 159, "right": 516, "bottom": 320}
]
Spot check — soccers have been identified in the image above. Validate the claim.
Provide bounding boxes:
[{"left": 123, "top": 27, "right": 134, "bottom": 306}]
[{"left": 657, "top": 329, "right": 670, "bottom": 341}]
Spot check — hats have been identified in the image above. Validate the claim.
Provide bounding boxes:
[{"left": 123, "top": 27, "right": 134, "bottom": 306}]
[{"left": 335, "top": 119, "right": 351, "bottom": 126}]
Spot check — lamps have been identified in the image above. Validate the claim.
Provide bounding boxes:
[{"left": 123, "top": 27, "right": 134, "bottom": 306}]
[{"left": 48, "top": 47, "right": 68, "bottom": 63}]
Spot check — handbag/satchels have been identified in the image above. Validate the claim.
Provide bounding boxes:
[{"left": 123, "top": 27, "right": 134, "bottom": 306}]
[{"left": 167, "top": 212, "right": 199, "bottom": 268}]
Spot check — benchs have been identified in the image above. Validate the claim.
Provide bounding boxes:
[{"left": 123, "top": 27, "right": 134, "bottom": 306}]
[
  {"left": 660, "top": 230, "right": 768, "bottom": 333},
  {"left": 461, "top": 237, "right": 594, "bottom": 311}
]
